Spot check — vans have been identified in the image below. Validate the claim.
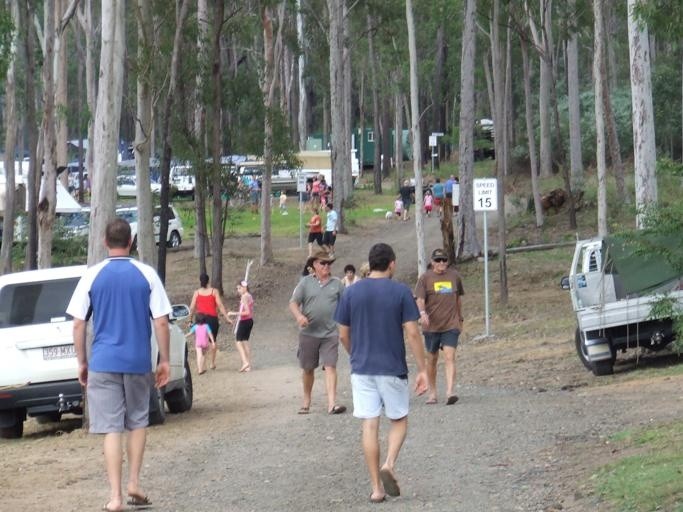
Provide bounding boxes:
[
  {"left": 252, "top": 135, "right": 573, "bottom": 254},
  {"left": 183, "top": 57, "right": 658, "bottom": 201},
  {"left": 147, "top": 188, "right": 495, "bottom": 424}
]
[{"left": 474, "top": 118, "right": 494, "bottom": 160}]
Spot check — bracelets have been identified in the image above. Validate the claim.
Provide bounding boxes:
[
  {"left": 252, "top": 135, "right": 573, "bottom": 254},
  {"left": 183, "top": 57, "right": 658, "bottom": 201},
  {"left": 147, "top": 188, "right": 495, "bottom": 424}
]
[
  {"left": 189, "top": 321, "right": 192, "bottom": 322},
  {"left": 459, "top": 320, "right": 464, "bottom": 322},
  {"left": 419, "top": 311, "right": 425, "bottom": 315}
]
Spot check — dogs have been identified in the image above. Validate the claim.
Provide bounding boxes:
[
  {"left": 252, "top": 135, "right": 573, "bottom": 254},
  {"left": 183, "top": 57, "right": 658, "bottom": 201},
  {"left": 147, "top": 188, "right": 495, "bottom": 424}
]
[{"left": 385, "top": 210, "right": 394, "bottom": 222}]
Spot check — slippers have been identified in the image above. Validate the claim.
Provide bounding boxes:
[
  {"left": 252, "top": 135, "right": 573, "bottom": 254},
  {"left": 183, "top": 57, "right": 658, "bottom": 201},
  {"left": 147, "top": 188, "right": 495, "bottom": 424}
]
[
  {"left": 125, "top": 493, "right": 152, "bottom": 506},
  {"left": 378, "top": 465, "right": 401, "bottom": 498},
  {"left": 326, "top": 404, "right": 346, "bottom": 415},
  {"left": 425, "top": 398, "right": 437, "bottom": 405},
  {"left": 297, "top": 407, "right": 309, "bottom": 414},
  {"left": 368, "top": 491, "right": 386, "bottom": 504},
  {"left": 197, "top": 364, "right": 216, "bottom": 376},
  {"left": 100, "top": 498, "right": 124, "bottom": 511},
  {"left": 444, "top": 393, "right": 459, "bottom": 406}
]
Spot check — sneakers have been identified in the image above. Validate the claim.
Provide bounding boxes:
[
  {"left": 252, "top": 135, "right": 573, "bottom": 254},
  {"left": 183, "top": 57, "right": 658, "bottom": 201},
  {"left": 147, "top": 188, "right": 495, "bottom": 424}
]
[{"left": 239, "top": 362, "right": 252, "bottom": 373}]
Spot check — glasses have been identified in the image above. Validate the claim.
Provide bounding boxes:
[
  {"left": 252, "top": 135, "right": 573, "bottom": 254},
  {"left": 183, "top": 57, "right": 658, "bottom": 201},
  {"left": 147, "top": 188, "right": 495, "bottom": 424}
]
[
  {"left": 314, "top": 260, "right": 331, "bottom": 266},
  {"left": 431, "top": 258, "right": 447, "bottom": 263}
]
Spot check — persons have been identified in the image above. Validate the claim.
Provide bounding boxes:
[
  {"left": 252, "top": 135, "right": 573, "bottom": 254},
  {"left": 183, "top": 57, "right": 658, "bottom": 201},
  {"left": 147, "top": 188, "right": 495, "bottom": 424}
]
[
  {"left": 289, "top": 244, "right": 346, "bottom": 415},
  {"left": 188, "top": 274, "right": 233, "bottom": 371},
  {"left": 249, "top": 174, "right": 261, "bottom": 214},
  {"left": 185, "top": 312, "right": 215, "bottom": 374},
  {"left": 395, "top": 174, "right": 460, "bottom": 221},
  {"left": 227, "top": 280, "right": 253, "bottom": 372},
  {"left": 334, "top": 243, "right": 427, "bottom": 502},
  {"left": 66, "top": 220, "right": 173, "bottom": 512},
  {"left": 279, "top": 190, "right": 289, "bottom": 215},
  {"left": 297, "top": 175, "right": 372, "bottom": 288},
  {"left": 413, "top": 249, "right": 464, "bottom": 404}
]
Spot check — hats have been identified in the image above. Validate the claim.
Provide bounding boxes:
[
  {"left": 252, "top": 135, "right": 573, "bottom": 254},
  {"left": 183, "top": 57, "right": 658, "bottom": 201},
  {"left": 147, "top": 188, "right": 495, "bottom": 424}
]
[
  {"left": 304, "top": 245, "right": 337, "bottom": 269},
  {"left": 430, "top": 248, "right": 449, "bottom": 261}
]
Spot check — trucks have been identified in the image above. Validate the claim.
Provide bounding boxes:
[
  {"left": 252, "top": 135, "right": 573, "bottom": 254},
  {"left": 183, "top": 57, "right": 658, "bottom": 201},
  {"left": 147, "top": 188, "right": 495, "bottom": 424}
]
[{"left": 561, "top": 231, "right": 681, "bottom": 376}]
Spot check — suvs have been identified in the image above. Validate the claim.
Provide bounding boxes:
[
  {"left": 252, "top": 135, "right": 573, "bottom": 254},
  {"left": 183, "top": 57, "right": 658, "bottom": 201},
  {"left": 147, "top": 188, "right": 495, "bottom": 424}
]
[
  {"left": 0, "top": 256, "right": 198, "bottom": 440},
  {"left": 115, "top": 205, "right": 184, "bottom": 249}
]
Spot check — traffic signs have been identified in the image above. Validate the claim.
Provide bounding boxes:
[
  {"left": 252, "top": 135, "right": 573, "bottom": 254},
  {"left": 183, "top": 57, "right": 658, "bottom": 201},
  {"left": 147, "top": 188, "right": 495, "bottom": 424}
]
[{"left": 473, "top": 178, "right": 498, "bottom": 212}]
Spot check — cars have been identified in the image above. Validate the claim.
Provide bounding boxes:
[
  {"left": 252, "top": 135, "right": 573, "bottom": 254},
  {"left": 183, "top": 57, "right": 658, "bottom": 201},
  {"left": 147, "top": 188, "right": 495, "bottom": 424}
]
[
  {"left": 65, "top": 159, "right": 298, "bottom": 202},
  {"left": 54, "top": 209, "right": 91, "bottom": 249}
]
